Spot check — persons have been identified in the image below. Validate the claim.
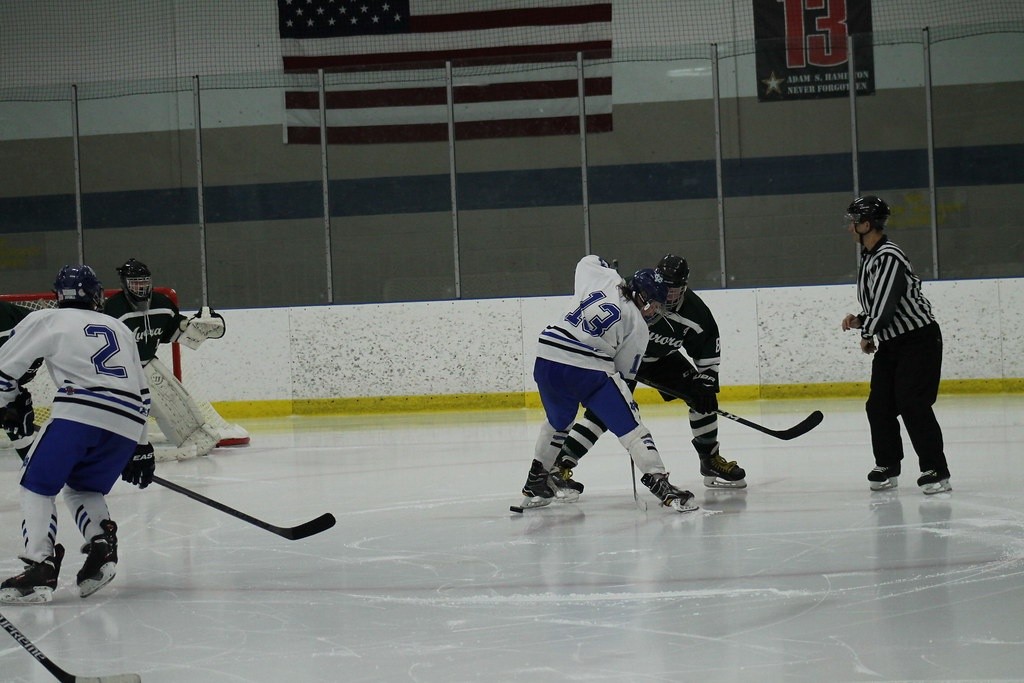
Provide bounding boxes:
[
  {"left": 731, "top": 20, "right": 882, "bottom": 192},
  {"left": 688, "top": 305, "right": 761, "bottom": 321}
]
[
  {"left": 0, "top": 301, "right": 45, "bottom": 463},
  {"left": 546, "top": 254, "right": 746, "bottom": 494},
  {"left": 103, "top": 258, "right": 226, "bottom": 461},
  {"left": 522, "top": 255, "right": 700, "bottom": 507},
  {"left": 842, "top": 195, "right": 951, "bottom": 486},
  {"left": 0, "top": 262, "right": 156, "bottom": 596}
]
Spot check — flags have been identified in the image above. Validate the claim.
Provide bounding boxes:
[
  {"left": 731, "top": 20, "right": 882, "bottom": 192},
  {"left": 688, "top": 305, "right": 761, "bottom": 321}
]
[{"left": 276, "top": 0, "right": 613, "bottom": 146}]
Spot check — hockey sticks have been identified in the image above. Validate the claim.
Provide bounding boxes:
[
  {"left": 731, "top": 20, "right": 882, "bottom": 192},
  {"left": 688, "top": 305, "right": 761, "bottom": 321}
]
[
  {"left": 630, "top": 457, "right": 649, "bottom": 512},
  {"left": 634, "top": 375, "right": 825, "bottom": 441},
  {"left": 0, "top": 612, "right": 142, "bottom": 683},
  {"left": 32, "top": 422, "right": 337, "bottom": 542}
]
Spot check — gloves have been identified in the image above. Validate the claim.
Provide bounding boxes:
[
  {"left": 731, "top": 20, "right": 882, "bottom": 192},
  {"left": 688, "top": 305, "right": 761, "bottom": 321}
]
[
  {"left": 686, "top": 388, "right": 718, "bottom": 415},
  {"left": 121, "top": 442, "right": 156, "bottom": 490}
]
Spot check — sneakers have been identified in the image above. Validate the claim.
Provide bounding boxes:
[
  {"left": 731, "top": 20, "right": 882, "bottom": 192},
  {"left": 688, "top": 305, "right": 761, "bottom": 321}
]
[
  {"left": 0, "top": 543, "right": 65, "bottom": 604},
  {"left": 640, "top": 472, "right": 699, "bottom": 511},
  {"left": 699, "top": 452, "right": 748, "bottom": 489},
  {"left": 521, "top": 458, "right": 554, "bottom": 508},
  {"left": 867, "top": 461, "right": 901, "bottom": 491},
  {"left": 546, "top": 453, "right": 584, "bottom": 503},
  {"left": 917, "top": 461, "right": 952, "bottom": 495},
  {"left": 77, "top": 520, "right": 119, "bottom": 598}
]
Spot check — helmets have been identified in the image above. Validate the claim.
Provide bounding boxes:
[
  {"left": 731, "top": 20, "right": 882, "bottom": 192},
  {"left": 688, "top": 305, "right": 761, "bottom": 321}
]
[
  {"left": 655, "top": 253, "right": 690, "bottom": 309},
  {"left": 118, "top": 259, "right": 153, "bottom": 301},
  {"left": 55, "top": 263, "right": 104, "bottom": 307},
  {"left": 843, "top": 194, "right": 891, "bottom": 225},
  {"left": 633, "top": 269, "right": 671, "bottom": 324}
]
